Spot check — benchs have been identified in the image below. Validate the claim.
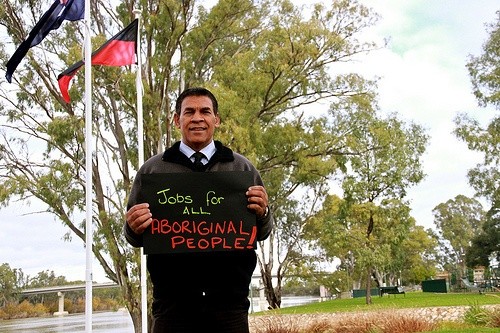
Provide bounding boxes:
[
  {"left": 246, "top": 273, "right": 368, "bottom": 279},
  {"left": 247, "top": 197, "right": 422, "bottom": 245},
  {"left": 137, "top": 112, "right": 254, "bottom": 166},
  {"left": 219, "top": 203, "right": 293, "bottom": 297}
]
[{"left": 380, "top": 285, "right": 406, "bottom": 299}]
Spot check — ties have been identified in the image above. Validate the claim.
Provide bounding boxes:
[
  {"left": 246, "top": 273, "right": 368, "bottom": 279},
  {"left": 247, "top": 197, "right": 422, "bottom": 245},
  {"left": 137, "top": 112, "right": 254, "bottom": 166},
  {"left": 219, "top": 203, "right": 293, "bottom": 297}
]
[{"left": 191, "top": 152, "right": 207, "bottom": 166}]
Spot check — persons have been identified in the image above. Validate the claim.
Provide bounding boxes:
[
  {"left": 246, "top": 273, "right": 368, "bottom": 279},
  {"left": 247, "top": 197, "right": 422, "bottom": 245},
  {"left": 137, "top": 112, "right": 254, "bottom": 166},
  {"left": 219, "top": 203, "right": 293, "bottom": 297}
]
[{"left": 123, "top": 87, "right": 273, "bottom": 333}]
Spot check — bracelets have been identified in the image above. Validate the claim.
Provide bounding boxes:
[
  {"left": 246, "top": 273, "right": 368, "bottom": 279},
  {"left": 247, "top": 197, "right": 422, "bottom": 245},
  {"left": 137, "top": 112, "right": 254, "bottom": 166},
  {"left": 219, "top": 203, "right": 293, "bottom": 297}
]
[{"left": 259, "top": 206, "right": 269, "bottom": 219}]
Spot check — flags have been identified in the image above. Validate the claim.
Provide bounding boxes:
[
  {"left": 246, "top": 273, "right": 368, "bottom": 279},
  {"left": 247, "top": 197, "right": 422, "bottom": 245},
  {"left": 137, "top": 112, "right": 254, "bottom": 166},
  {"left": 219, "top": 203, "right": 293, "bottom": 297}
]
[
  {"left": 5, "top": 0, "right": 85, "bottom": 84},
  {"left": 57, "top": 18, "right": 138, "bottom": 105}
]
